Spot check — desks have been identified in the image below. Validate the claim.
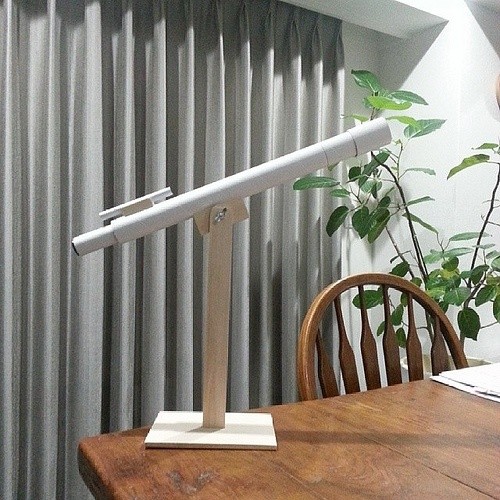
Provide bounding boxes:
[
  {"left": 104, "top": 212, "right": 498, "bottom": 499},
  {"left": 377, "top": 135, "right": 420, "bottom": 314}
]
[{"left": 76, "top": 363, "right": 500, "bottom": 500}]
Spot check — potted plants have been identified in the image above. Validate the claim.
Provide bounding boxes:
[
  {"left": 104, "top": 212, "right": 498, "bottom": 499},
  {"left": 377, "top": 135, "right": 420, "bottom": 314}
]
[{"left": 289, "top": 70, "right": 500, "bottom": 381}]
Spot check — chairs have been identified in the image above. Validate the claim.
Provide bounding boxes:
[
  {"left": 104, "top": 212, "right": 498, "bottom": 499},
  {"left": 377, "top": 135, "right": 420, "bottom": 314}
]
[{"left": 297, "top": 274, "right": 469, "bottom": 401}]
